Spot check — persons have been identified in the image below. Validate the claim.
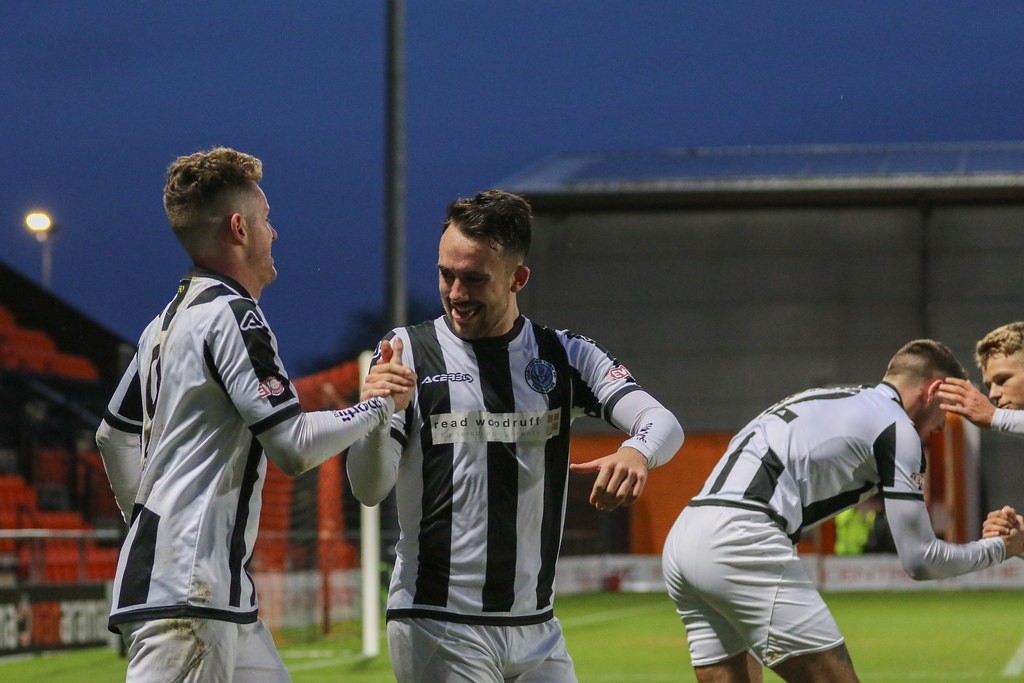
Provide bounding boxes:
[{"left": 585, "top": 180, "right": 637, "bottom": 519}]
[
  {"left": 95, "top": 147, "right": 418, "bottom": 683},
  {"left": 346, "top": 188, "right": 685, "bottom": 683},
  {"left": 662, "top": 322, "right": 1024, "bottom": 683}
]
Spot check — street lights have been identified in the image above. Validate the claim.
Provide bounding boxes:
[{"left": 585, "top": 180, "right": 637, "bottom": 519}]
[{"left": 25, "top": 212, "right": 58, "bottom": 286}]
